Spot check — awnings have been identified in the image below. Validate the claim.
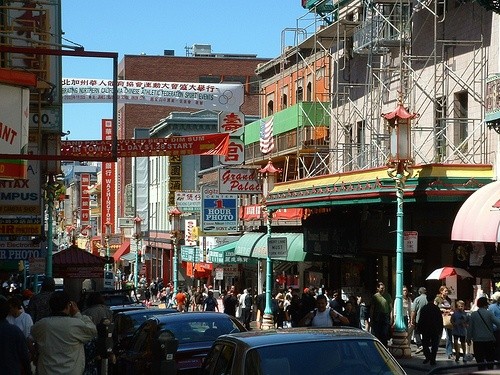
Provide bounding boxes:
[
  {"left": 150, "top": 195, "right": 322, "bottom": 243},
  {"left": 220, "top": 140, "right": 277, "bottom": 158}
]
[
  {"left": 119, "top": 252, "right": 136, "bottom": 263},
  {"left": 209, "top": 240, "right": 258, "bottom": 265},
  {"left": 112, "top": 241, "right": 131, "bottom": 263},
  {"left": 451, "top": 180, "right": 500, "bottom": 253},
  {"left": 234, "top": 231, "right": 308, "bottom": 262}
]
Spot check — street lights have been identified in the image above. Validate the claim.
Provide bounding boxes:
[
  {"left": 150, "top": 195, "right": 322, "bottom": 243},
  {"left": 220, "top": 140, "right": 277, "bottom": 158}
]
[
  {"left": 132, "top": 213, "right": 144, "bottom": 295},
  {"left": 166, "top": 200, "right": 182, "bottom": 297},
  {"left": 37, "top": 120, "right": 70, "bottom": 278},
  {"left": 105, "top": 220, "right": 115, "bottom": 271},
  {"left": 256, "top": 151, "right": 284, "bottom": 315},
  {"left": 380, "top": 94, "right": 421, "bottom": 332}
]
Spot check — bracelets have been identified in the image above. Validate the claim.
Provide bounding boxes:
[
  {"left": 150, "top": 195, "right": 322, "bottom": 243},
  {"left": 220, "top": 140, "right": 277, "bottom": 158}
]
[{"left": 335, "top": 314, "right": 340, "bottom": 319}]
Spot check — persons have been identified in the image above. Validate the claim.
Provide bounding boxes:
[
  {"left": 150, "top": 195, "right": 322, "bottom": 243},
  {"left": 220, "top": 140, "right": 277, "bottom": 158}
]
[
  {"left": 297, "top": 294, "right": 349, "bottom": 327},
  {"left": 370, "top": 282, "right": 394, "bottom": 349},
  {"left": 0, "top": 271, "right": 500, "bottom": 375},
  {"left": 417, "top": 291, "right": 443, "bottom": 366},
  {"left": 29, "top": 289, "right": 98, "bottom": 375},
  {"left": 466, "top": 296, "right": 500, "bottom": 371}
]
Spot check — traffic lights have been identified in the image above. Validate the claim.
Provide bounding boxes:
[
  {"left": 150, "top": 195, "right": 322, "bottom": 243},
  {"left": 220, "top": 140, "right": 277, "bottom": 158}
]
[
  {"left": 18, "top": 260, "right": 24, "bottom": 272},
  {"left": 23, "top": 260, "right": 30, "bottom": 272}
]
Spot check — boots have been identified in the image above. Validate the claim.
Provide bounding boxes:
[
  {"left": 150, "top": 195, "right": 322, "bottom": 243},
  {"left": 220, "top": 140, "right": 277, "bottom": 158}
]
[
  {"left": 431, "top": 354, "right": 438, "bottom": 366},
  {"left": 423, "top": 353, "right": 430, "bottom": 364}
]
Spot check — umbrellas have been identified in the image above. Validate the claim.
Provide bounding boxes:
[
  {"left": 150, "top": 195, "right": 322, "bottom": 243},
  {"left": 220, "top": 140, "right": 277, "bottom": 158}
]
[{"left": 426, "top": 266, "right": 473, "bottom": 282}]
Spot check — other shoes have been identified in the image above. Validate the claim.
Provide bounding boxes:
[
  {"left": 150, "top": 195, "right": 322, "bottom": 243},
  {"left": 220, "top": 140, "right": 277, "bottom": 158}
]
[
  {"left": 463, "top": 358, "right": 468, "bottom": 363},
  {"left": 415, "top": 346, "right": 423, "bottom": 354},
  {"left": 455, "top": 359, "right": 459, "bottom": 362},
  {"left": 245, "top": 325, "right": 253, "bottom": 331}
]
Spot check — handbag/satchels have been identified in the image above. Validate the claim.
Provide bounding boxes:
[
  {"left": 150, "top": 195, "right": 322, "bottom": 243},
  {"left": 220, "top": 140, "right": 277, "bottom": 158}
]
[{"left": 493, "top": 331, "right": 500, "bottom": 340}]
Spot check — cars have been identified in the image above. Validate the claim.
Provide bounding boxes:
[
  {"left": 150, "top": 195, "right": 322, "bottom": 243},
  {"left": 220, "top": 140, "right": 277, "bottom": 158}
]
[
  {"left": 198, "top": 327, "right": 407, "bottom": 375},
  {"left": 110, "top": 313, "right": 248, "bottom": 375},
  {"left": 107, "top": 304, "right": 182, "bottom": 352}
]
